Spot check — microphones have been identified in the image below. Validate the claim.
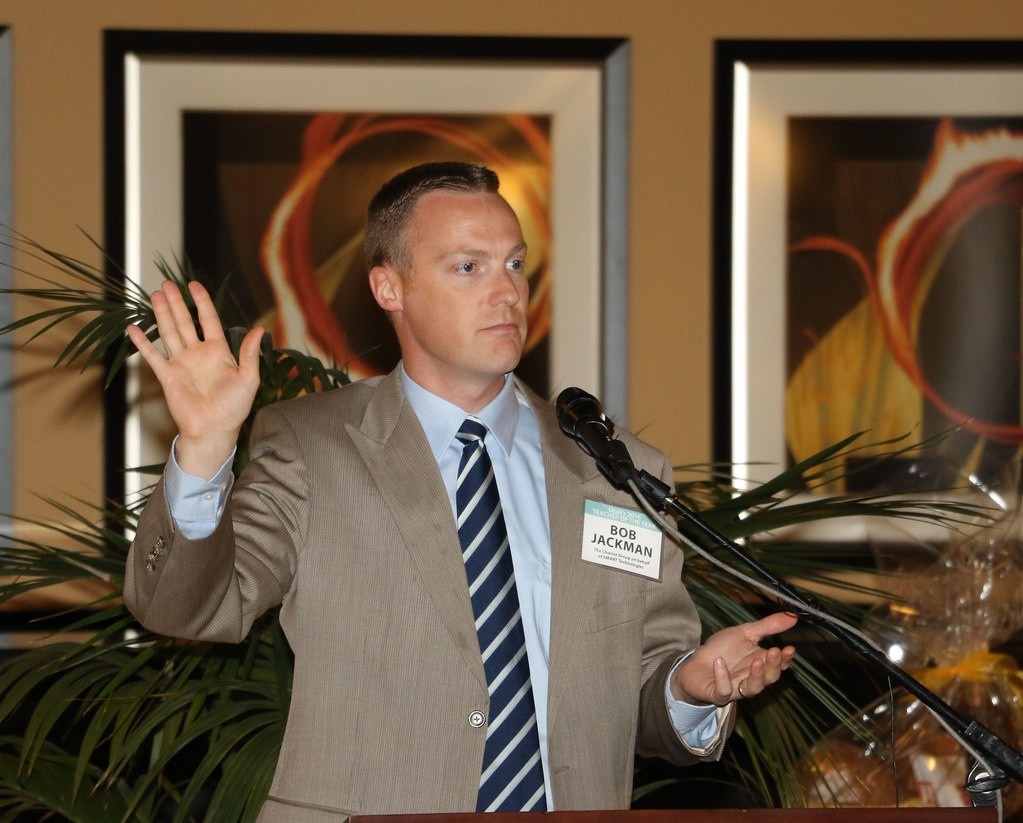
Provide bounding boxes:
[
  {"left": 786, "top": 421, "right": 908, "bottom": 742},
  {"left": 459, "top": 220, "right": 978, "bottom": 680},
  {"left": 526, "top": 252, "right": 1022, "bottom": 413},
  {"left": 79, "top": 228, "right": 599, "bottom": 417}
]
[{"left": 555, "top": 386, "right": 635, "bottom": 486}]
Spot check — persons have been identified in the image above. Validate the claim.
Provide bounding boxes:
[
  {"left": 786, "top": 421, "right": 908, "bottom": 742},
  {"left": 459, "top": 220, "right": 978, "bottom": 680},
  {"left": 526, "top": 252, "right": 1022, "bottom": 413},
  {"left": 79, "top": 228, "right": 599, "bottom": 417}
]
[{"left": 123, "top": 161, "right": 798, "bottom": 823}]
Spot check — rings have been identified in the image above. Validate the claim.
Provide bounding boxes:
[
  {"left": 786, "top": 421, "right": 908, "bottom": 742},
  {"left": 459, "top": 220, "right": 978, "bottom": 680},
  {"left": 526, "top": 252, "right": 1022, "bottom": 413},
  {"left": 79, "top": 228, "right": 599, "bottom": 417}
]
[{"left": 738, "top": 680, "right": 746, "bottom": 698}]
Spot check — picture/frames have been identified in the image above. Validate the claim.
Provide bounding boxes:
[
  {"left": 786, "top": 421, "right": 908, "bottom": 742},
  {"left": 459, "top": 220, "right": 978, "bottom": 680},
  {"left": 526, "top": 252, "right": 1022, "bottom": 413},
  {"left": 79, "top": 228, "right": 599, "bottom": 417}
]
[
  {"left": 101, "top": 25, "right": 630, "bottom": 578},
  {"left": 712, "top": 35, "right": 1023, "bottom": 574}
]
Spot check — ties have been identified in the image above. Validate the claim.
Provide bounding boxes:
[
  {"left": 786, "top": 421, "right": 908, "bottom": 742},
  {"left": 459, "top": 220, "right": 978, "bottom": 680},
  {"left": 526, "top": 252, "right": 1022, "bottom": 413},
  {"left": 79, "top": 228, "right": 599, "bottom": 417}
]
[{"left": 455, "top": 418, "right": 547, "bottom": 813}]
